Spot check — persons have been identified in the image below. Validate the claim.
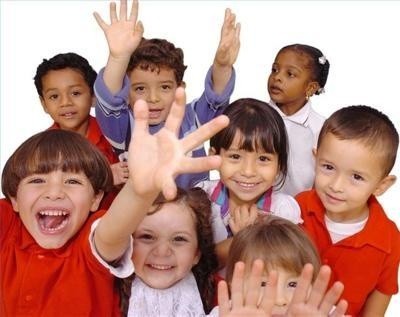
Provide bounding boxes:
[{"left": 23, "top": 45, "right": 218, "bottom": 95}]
[
  {"left": 205, "top": 216, "right": 350, "bottom": 317},
  {"left": 31, "top": 50, "right": 129, "bottom": 211},
  {"left": 119, "top": 186, "right": 230, "bottom": 317},
  {"left": 91, "top": 0, "right": 242, "bottom": 191},
  {"left": 3, "top": 85, "right": 230, "bottom": 317},
  {"left": 265, "top": 43, "right": 331, "bottom": 201},
  {"left": 194, "top": 97, "right": 306, "bottom": 309},
  {"left": 294, "top": 103, "right": 400, "bottom": 317}
]
